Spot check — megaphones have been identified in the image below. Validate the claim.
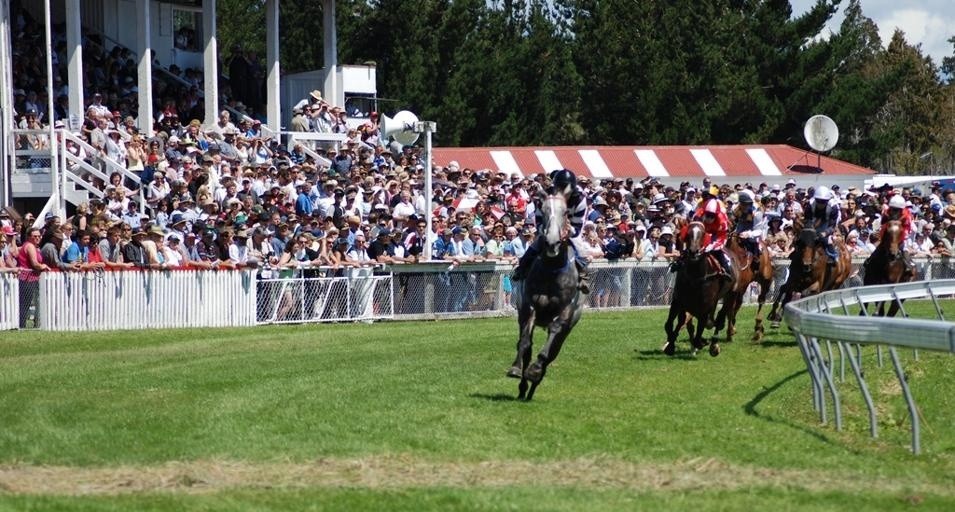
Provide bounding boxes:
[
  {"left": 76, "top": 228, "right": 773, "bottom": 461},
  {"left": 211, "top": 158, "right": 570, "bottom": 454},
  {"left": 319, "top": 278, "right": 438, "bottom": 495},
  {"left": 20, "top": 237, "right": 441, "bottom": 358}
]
[
  {"left": 380, "top": 114, "right": 405, "bottom": 140},
  {"left": 393, "top": 109, "right": 420, "bottom": 145}
]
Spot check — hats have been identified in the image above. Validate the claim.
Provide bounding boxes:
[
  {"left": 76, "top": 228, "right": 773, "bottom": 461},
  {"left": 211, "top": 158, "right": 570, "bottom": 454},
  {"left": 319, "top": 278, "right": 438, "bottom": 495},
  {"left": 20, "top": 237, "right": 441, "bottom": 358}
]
[
  {"left": 0, "top": 76, "right": 424, "bottom": 244},
  {"left": 431, "top": 160, "right": 551, "bottom": 242},
  {"left": 892, "top": 180, "right": 955, "bottom": 249},
  {"left": 576, "top": 172, "right": 892, "bottom": 247}
]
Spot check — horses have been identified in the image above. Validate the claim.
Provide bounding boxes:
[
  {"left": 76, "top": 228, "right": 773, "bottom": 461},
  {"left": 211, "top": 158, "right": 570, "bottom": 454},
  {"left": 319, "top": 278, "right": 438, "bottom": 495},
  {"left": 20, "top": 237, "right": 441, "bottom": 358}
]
[
  {"left": 858, "top": 212, "right": 914, "bottom": 317},
  {"left": 663, "top": 216, "right": 774, "bottom": 358},
  {"left": 767, "top": 217, "right": 852, "bottom": 329},
  {"left": 506, "top": 183, "right": 585, "bottom": 401}
]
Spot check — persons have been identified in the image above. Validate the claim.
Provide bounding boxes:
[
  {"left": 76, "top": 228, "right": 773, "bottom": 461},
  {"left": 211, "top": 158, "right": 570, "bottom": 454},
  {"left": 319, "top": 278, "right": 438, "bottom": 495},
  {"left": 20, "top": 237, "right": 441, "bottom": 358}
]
[{"left": 0, "top": 5, "right": 955, "bottom": 327}]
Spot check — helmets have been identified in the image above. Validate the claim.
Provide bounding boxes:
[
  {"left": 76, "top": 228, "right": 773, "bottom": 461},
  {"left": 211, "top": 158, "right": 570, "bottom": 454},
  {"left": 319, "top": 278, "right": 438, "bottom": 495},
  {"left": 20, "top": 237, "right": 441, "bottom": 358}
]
[
  {"left": 888, "top": 194, "right": 906, "bottom": 209},
  {"left": 552, "top": 167, "right": 575, "bottom": 191}
]
